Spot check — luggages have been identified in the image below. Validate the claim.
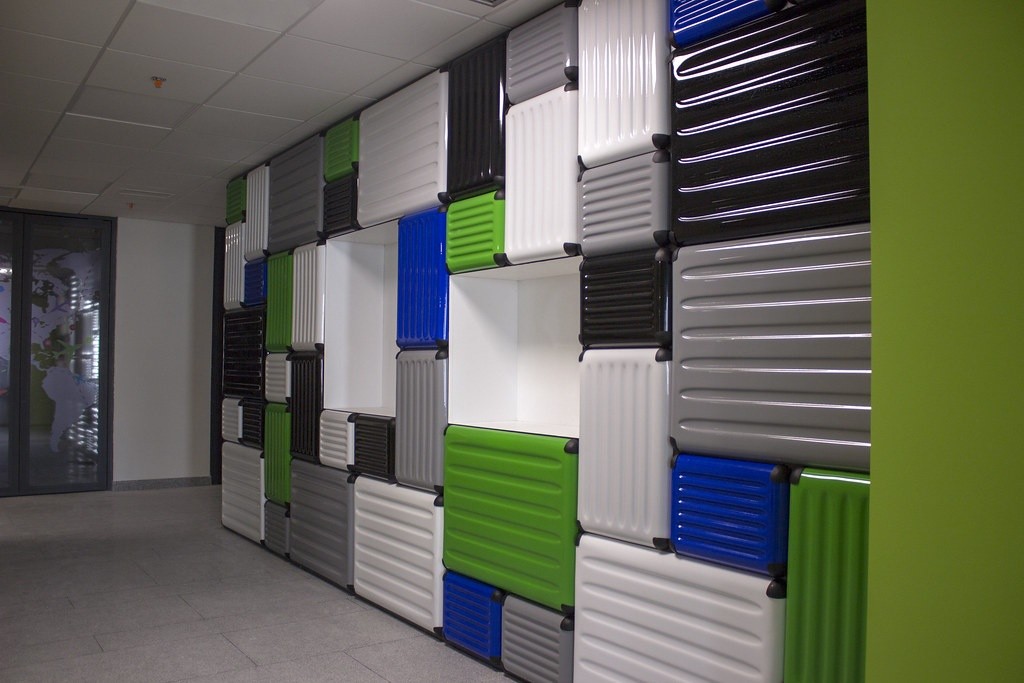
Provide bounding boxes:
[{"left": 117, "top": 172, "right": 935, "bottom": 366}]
[{"left": 219, "top": 2, "right": 869, "bottom": 683}]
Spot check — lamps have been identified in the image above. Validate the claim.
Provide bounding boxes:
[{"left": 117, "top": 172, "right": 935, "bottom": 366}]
[{"left": 151, "top": 77, "right": 167, "bottom": 89}]
